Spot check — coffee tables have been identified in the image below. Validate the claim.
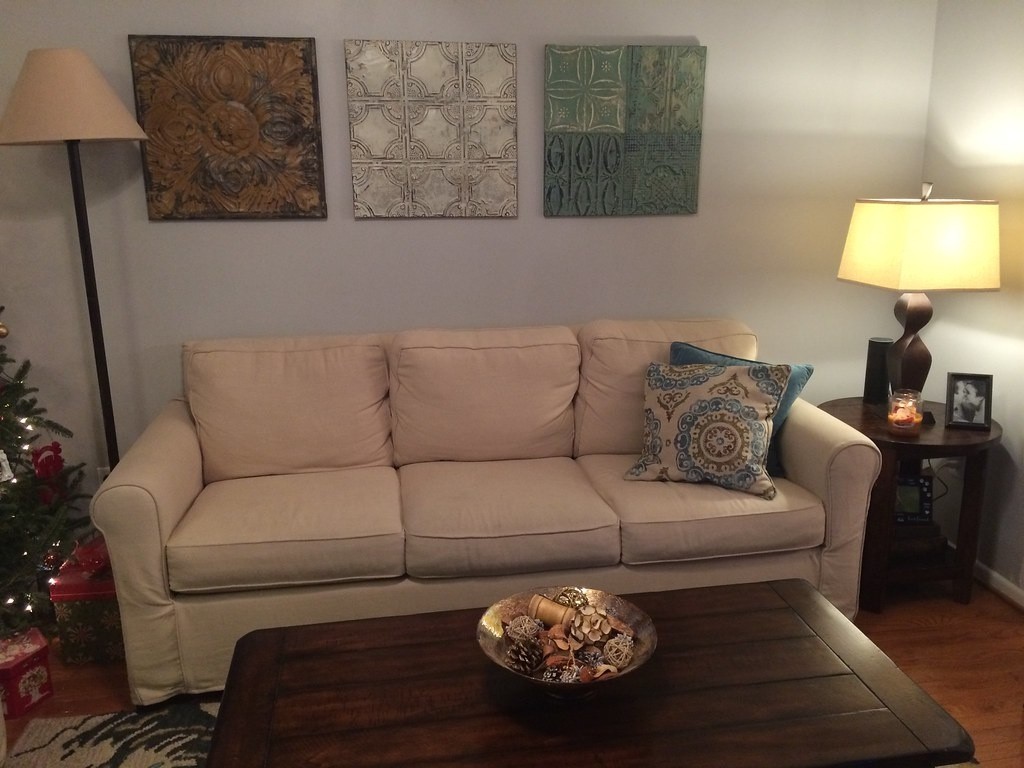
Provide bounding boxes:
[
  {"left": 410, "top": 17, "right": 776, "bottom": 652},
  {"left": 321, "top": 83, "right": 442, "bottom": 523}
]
[{"left": 208, "top": 577, "right": 976, "bottom": 768}]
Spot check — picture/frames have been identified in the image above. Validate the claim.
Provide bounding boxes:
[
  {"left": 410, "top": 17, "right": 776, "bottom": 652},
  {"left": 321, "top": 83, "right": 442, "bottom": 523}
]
[{"left": 944, "top": 370, "right": 994, "bottom": 432}]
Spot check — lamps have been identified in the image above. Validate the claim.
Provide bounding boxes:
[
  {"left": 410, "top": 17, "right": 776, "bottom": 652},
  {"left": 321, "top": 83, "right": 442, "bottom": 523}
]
[
  {"left": 0, "top": 47, "right": 151, "bottom": 473},
  {"left": 836, "top": 180, "right": 1002, "bottom": 427}
]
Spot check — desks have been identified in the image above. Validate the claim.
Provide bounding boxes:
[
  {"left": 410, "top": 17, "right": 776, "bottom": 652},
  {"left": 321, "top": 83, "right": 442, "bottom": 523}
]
[{"left": 817, "top": 397, "right": 1002, "bottom": 613}]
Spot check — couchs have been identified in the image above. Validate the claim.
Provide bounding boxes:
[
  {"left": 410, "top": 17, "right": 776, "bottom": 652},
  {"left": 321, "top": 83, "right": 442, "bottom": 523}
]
[{"left": 86, "top": 320, "right": 883, "bottom": 714}]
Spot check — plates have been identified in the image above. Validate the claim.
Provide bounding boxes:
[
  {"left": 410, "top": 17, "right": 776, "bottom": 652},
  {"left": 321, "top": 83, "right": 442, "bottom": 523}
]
[{"left": 477, "top": 585, "right": 658, "bottom": 697}]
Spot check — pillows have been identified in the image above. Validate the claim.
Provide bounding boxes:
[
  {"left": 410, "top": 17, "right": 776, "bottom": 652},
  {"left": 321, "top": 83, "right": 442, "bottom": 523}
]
[
  {"left": 668, "top": 339, "right": 814, "bottom": 475},
  {"left": 624, "top": 361, "right": 790, "bottom": 501}
]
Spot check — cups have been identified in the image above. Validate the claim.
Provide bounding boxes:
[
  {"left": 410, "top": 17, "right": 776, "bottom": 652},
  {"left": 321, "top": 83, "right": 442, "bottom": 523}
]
[
  {"left": 864, "top": 336, "right": 894, "bottom": 404},
  {"left": 887, "top": 389, "right": 923, "bottom": 436}
]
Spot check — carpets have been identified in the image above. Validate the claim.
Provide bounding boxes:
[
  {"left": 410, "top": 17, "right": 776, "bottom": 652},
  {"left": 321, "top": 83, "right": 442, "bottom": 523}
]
[{"left": 1, "top": 693, "right": 222, "bottom": 768}]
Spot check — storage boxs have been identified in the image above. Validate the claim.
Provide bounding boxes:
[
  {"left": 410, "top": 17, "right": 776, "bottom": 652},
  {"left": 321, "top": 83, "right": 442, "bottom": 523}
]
[{"left": 49, "top": 542, "right": 125, "bottom": 667}]
